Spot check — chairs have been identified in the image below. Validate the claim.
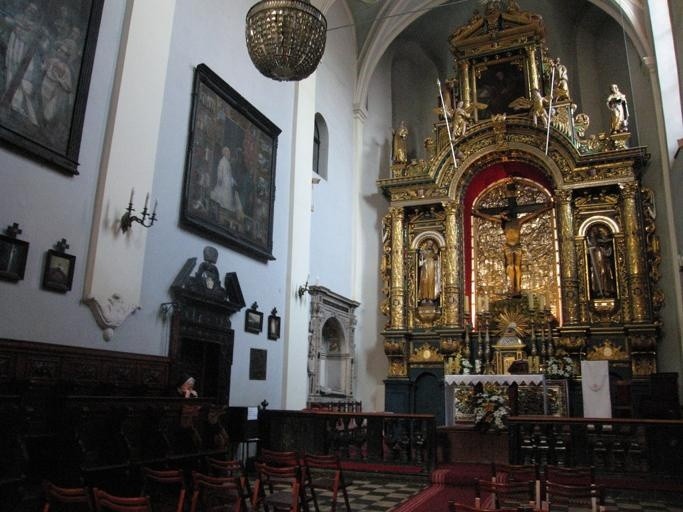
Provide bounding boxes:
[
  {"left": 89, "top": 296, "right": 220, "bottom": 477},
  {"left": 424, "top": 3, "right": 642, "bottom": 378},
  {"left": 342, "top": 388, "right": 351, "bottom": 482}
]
[
  {"left": 448, "top": 460, "right": 607, "bottom": 512},
  {"left": 41, "top": 447, "right": 353, "bottom": 512}
]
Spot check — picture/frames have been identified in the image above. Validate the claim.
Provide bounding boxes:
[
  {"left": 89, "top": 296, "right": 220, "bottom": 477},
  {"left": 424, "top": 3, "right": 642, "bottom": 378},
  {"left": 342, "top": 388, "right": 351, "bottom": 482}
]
[
  {"left": 245, "top": 309, "right": 263, "bottom": 332},
  {"left": 43, "top": 249, "right": 76, "bottom": 293},
  {"left": 0, "top": 0, "right": 104, "bottom": 174},
  {"left": 0, "top": 234, "right": 29, "bottom": 280},
  {"left": 268, "top": 315, "right": 280, "bottom": 338},
  {"left": 179, "top": 62, "right": 283, "bottom": 260}
]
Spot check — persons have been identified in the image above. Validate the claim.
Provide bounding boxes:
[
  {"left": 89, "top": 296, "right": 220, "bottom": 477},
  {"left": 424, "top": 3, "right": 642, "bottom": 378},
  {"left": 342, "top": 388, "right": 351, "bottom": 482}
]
[
  {"left": 210, "top": 145, "right": 236, "bottom": 211},
  {"left": 585, "top": 224, "right": 615, "bottom": 297},
  {"left": 418, "top": 239, "right": 438, "bottom": 305},
  {"left": 389, "top": 117, "right": 407, "bottom": 163},
  {"left": 471, "top": 201, "right": 556, "bottom": 295},
  {"left": 605, "top": 83, "right": 628, "bottom": 133},
  {"left": 169, "top": 370, "right": 198, "bottom": 399},
  {"left": 528, "top": 87, "right": 551, "bottom": 130},
  {"left": 0, "top": 1, "right": 82, "bottom": 134},
  {"left": 452, "top": 100, "right": 472, "bottom": 142},
  {"left": 548, "top": 56, "right": 571, "bottom": 102}
]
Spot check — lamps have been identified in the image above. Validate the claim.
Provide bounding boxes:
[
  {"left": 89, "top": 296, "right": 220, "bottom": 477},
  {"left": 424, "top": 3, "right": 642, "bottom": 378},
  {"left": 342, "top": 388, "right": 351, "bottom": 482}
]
[
  {"left": 245, "top": 0, "right": 328, "bottom": 82},
  {"left": 298, "top": 271, "right": 319, "bottom": 298},
  {"left": 120, "top": 185, "right": 159, "bottom": 234}
]
